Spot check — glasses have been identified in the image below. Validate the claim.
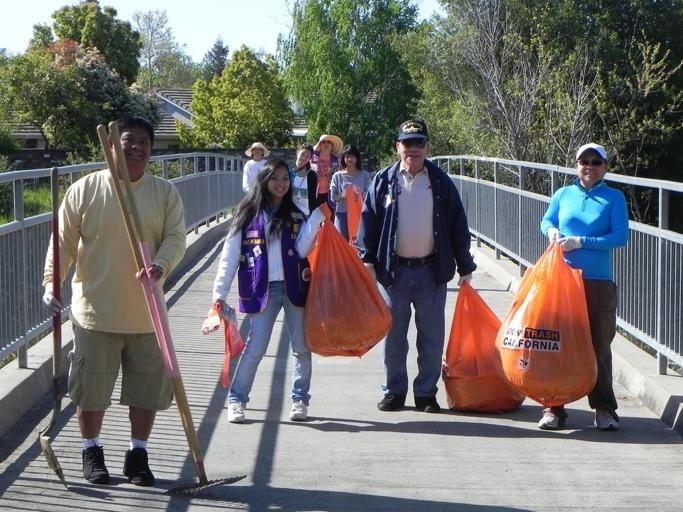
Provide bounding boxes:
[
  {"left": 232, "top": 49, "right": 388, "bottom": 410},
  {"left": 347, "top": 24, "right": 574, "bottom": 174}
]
[
  {"left": 578, "top": 159, "right": 602, "bottom": 166},
  {"left": 400, "top": 139, "right": 428, "bottom": 149}
]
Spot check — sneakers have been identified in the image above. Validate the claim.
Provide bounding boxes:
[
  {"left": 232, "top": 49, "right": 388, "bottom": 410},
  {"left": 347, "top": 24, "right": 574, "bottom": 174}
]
[
  {"left": 226, "top": 401, "right": 245, "bottom": 422},
  {"left": 377, "top": 393, "right": 406, "bottom": 411},
  {"left": 413, "top": 396, "right": 441, "bottom": 413},
  {"left": 81, "top": 445, "right": 109, "bottom": 484},
  {"left": 288, "top": 400, "right": 307, "bottom": 421},
  {"left": 593, "top": 408, "right": 619, "bottom": 431},
  {"left": 122, "top": 448, "right": 155, "bottom": 486},
  {"left": 537, "top": 412, "right": 567, "bottom": 430}
]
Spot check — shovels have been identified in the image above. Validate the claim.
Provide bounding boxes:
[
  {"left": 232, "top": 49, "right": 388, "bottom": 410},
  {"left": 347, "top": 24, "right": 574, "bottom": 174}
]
[{"left": 39, "top": 167, "right": 69, "bottom": 490}]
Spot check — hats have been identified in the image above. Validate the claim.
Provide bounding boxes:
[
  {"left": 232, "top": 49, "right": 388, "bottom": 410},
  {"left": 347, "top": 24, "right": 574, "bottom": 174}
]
[
  {"left": 244, "top": 142, "right": 270, "bottom": 158},
  {"left": 575, "top": 143, "right": 608, "bottom": 163},
  {"left": 397, "top": 120, "right": 429, "bottom": 141},
  {"left": 313, "top": 134, "right": 343, "bottom": 156}
]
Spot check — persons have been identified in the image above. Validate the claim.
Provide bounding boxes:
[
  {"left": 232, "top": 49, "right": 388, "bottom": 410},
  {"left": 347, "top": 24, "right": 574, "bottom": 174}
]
[
  {"left": 213, "top": 158, "right": 331, "bottom": 422},
  {"left": 538, "top": 141, "right": 630, "bottom": 430},
  {"left": 355, "top": 118, "right": 476, "bottom": 412},
  {"left": 242, "top": 135, "right": 373, "bottom": 249},
  {"left": 42, "top": 112, "right": 189, "bottom": 487}
]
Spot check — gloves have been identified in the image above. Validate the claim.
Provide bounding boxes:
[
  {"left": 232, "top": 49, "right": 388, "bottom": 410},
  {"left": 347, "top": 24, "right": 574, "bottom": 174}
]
[
  {"left": 362, "top": 263, "right": 377, "bottom": 285},
  {"left": 457, "top": 272, "right": 472, "bottom": 286},
  {"left": 546, "top": 227, "right": 581, "bottom": 252}
]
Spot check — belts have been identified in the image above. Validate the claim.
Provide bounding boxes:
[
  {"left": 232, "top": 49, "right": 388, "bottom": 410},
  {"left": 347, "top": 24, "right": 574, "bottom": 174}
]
[{"left": 392, "top": 254, "right": 435, "bottom": 267}]
